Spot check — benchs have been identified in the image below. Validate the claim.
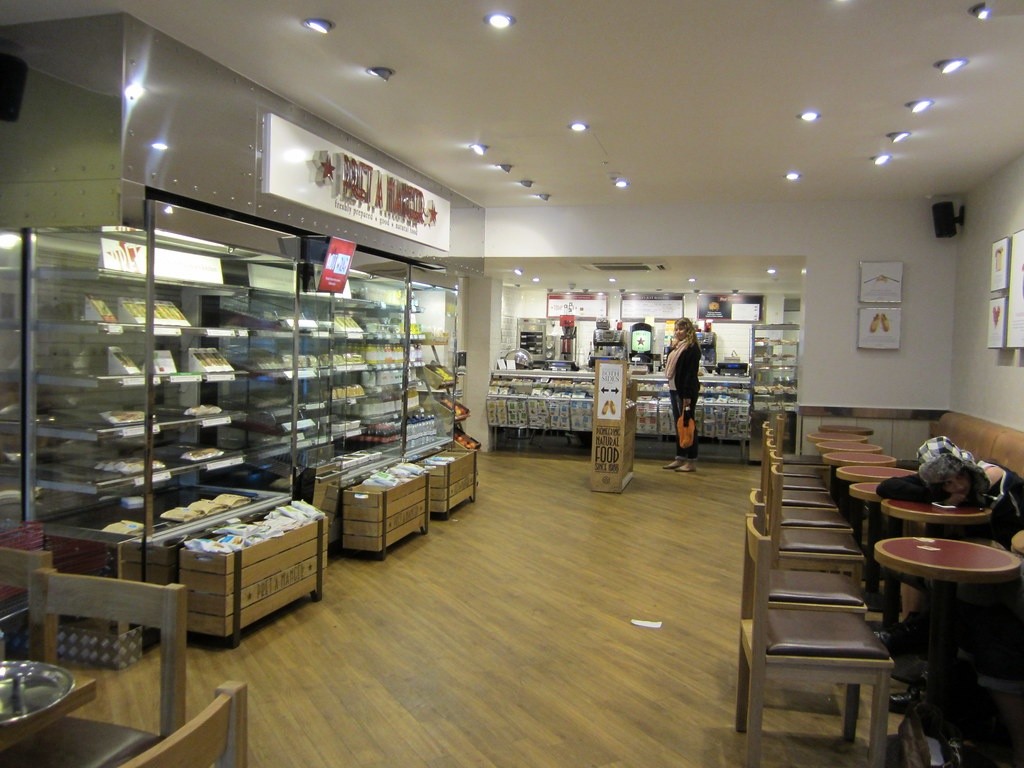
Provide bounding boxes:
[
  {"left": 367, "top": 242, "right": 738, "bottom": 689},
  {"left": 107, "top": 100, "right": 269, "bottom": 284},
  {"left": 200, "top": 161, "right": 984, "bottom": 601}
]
[{"left": 901, "top": 413, "right": 1024, "bottom": 768}]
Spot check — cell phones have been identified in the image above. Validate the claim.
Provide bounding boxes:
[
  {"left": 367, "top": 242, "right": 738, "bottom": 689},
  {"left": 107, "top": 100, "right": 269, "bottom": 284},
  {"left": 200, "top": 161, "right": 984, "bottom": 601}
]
[{"left": 932, "top": 502, "right": 956, "bottom": 509}]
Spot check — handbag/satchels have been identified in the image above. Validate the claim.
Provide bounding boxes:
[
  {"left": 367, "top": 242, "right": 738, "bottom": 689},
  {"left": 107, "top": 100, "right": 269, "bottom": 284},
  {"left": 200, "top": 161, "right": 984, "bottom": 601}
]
[
  {"left": 676, "top": 402, "right": 695, "bottom": 449},
  {"left": 897, "top": 701, "right": 964, "bottom": 768}
]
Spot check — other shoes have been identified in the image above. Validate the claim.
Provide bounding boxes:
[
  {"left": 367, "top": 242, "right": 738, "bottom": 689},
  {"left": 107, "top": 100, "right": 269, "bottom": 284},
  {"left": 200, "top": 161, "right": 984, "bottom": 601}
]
[
  {"left": 675, "top": 464, "right": 696, "bottom": 471},
  {"left": 663, "top": 460, "right": 681, "bottom": 469}
]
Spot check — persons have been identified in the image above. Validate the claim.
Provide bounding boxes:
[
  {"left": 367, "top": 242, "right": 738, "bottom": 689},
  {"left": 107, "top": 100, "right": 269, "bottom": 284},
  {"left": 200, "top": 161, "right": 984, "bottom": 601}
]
[
  {"left": 875, "top": 436, "right": 1024, "bottom": 716},
  {"left": 662, "top": 317, "right": 702, "bottom": 472}
]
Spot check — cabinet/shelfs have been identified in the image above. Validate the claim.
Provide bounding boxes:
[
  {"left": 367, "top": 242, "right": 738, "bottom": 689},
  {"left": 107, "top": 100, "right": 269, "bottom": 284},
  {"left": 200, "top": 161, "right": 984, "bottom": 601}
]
[
  {"left": 749, "top": 323, "right": 802, "bottom": 413},
  {"left": 20, "top": 201, "right": 461, "bottom": 545}
]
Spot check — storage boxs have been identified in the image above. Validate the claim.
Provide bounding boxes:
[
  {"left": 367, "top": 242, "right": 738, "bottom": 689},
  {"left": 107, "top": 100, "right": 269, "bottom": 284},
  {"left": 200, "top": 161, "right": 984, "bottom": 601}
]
[
  {"left": 178, "top": 515, "right": 330, "bottom": 651},
  {"left": 415, "top": 448, "right": 477, "bottom": 521},
  {"left": 340, "top": 468, "right": 431, "bottom": 562}
]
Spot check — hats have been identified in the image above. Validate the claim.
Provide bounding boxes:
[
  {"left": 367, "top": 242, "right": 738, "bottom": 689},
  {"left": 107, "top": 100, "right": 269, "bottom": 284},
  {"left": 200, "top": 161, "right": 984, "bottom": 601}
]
[{"left": 916, "top": 436, "right": 990, "bottom": 503}]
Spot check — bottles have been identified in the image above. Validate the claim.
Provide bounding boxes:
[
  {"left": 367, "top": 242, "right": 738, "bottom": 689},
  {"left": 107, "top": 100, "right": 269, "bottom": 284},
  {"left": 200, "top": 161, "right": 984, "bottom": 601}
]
[{"left": 329, "top": 343, "right": 437, "bottom": 450}]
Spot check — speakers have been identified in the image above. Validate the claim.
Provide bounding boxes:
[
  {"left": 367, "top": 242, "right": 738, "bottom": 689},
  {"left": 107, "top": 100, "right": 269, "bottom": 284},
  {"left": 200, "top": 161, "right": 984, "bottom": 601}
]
[
  {"left": 0, "top": 52, "right": 28, "bottom": 123},
  {"left": 932, "top": 201, "right": 957, "bottom": 238}
]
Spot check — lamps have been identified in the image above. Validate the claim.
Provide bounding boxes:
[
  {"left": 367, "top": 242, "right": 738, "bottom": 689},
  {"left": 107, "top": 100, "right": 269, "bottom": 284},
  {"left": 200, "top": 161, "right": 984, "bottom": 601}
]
[
  {"left": 300, "top": 20, "right": 550, "bottom": 207},
  {"left": 872, "top": 4, "right": 989, "bottom": 169}
]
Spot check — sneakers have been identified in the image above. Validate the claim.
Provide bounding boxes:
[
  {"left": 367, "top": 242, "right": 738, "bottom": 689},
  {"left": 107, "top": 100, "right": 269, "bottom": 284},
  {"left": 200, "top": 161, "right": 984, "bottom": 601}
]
[
  {"left": 888, "top": 671, "right": 928, "bottom": 714},
  {"left": 873, "top": 611, "right": 930, "bottom": 654}
]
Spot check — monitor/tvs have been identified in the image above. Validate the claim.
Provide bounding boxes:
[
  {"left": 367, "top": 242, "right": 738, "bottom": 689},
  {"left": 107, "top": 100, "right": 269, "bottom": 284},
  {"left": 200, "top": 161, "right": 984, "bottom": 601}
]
[{"left": 546, "top": 359, "right": 578, "bottom": 372}]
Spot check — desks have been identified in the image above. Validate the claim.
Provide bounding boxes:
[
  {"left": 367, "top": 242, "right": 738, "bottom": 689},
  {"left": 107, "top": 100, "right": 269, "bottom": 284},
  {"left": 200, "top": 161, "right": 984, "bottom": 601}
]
[
  {"left": 0, "top": 715, "right": 164, "bottom": 768},
  {"left": 804, "top": 424, "right": 1024, "bottom": 742}
]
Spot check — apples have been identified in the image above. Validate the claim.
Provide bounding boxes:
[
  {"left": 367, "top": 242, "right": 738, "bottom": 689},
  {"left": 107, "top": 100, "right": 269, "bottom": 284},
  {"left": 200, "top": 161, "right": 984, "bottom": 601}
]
[
  {"left": 441, "top": 399, "right": 465, "bottom": 416},
  {"left": 454, "top": 433, "right": 477, "bottom": 450}
]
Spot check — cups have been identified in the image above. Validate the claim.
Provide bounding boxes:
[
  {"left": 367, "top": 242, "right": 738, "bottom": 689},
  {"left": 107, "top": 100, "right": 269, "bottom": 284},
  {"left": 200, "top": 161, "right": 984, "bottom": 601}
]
[
  {"left": 617, "top": 322, "right": 622, "bottom": 330},
  {"left": 704, "top": 323, "right": 711, "bottom": 332}
]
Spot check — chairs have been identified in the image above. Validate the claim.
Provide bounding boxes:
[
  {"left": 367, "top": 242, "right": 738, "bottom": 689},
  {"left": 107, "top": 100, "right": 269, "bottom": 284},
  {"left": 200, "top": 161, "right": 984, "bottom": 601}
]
[
  {"left": 119, "top": 679, "right": 248, "bottom": 768},
  {"left": 734, "top": 411, "right": 896, "bottom": 768},
  {"left": 26, "top": 568, "right": 187, "bottom": 736}
]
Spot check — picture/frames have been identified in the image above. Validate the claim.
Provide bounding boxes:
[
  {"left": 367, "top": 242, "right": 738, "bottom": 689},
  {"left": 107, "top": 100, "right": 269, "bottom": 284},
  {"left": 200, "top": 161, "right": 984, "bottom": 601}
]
[
  {"left": 986, "top": 296, "right": 1015, "bottom": 352},
  {"left": 859, "top": 308, "right": 903, "bottom": 349},
  {"left": 858, "top": 261, "right": 905, "bottom": 304},
  {"left": 1005, "top": 228, "right": 1024, "bottom": 350},
  {"left": 990, "top": 236, "right": 1012, "bottom": 293}
]
